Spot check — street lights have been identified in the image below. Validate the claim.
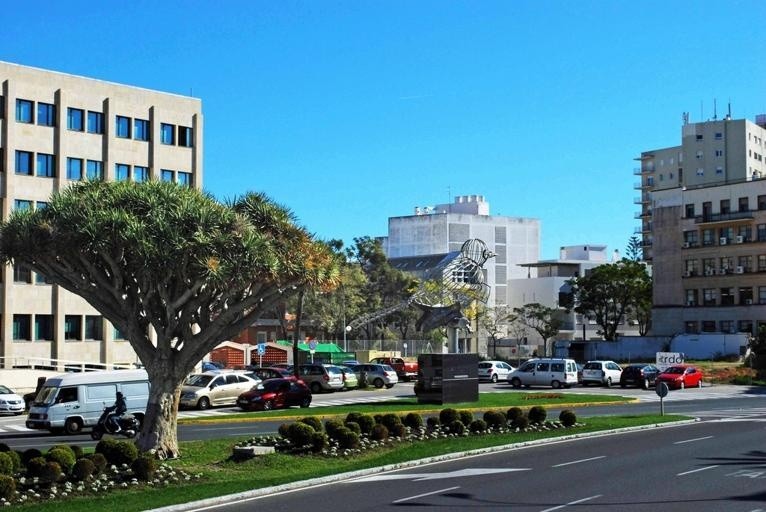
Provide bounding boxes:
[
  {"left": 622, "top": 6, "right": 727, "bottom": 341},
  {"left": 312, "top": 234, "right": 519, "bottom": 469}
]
[{"left": 342, "top": 325, "right": 352, "bottom": 353}]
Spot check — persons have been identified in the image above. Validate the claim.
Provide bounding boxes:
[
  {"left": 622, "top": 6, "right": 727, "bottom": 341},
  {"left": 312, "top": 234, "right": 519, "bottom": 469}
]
[{"left": 110, "top": 392, "right": 127, "bottom": 434}]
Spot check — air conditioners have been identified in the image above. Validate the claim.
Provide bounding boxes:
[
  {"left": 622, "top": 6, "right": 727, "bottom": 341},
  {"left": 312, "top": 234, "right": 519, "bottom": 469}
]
[
  {"left": 682, "top": 271, "right": 689, "bottom": 276},
  {"left": 706, "top": 269, "right": 711, "bottom": 274},
  {"left": 719, "top": 237, "right": 726, "bottom": 244},
  {"left": 682, "top": 301, "right": 689, "bottom": 307},
  {"left": 681, "top": 241, "right": 689, "bottom": 248},
  {"left": 718, "top": 268, "right": 724, "bottom": 273},
  {"left": 736, "top": 235, "right": 742, "bottom": 242},
  {"left": 736, "top": 266, "right": 742, "bottom": 272},
  {"left": 746, "top": 299, "right": 752, "bottom": 304}
]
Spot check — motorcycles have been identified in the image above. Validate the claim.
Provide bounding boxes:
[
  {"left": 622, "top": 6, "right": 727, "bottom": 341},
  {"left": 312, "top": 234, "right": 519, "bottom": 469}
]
[{"left": 91, "top": 403, "right": 137, "bottom": 441}]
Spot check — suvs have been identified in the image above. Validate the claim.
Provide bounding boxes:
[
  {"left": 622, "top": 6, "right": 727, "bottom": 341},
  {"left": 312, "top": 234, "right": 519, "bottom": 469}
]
[
  {"left": 582, "top": 361, "right": 623, "bottom": 387},
  {"left": 22, "top": 377, "right": 46, "bottom": 410}
]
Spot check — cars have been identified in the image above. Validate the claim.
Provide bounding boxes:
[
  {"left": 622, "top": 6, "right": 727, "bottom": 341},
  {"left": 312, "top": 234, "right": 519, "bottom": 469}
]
[
  {"left": 177, "top": 358, "right": 418, "bottom": 411},
  {"left": 477, "top": 361, "right": 515, "bottom": 382},
  {"left": 655, "top": 365, "right": 703, "bottom": 391},
  {"left": 560, "top": 363, "right": 582, "bottom": 383},
  {"left": 620, "top": 365, "right": 660, "bottom": 389},
  {"left": 0, "top": 385, "right": 25, "bottom": 416}
]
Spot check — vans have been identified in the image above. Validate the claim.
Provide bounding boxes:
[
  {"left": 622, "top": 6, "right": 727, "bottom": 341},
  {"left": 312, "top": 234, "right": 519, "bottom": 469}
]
[{"left": 506, "top": 358, "right": 578, "bottom": 390}]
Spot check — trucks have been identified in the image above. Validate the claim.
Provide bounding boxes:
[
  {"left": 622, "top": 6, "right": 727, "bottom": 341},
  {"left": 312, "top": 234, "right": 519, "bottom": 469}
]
[{"left": 25, "top": 369, "right": 151, "bottom": 434}]
